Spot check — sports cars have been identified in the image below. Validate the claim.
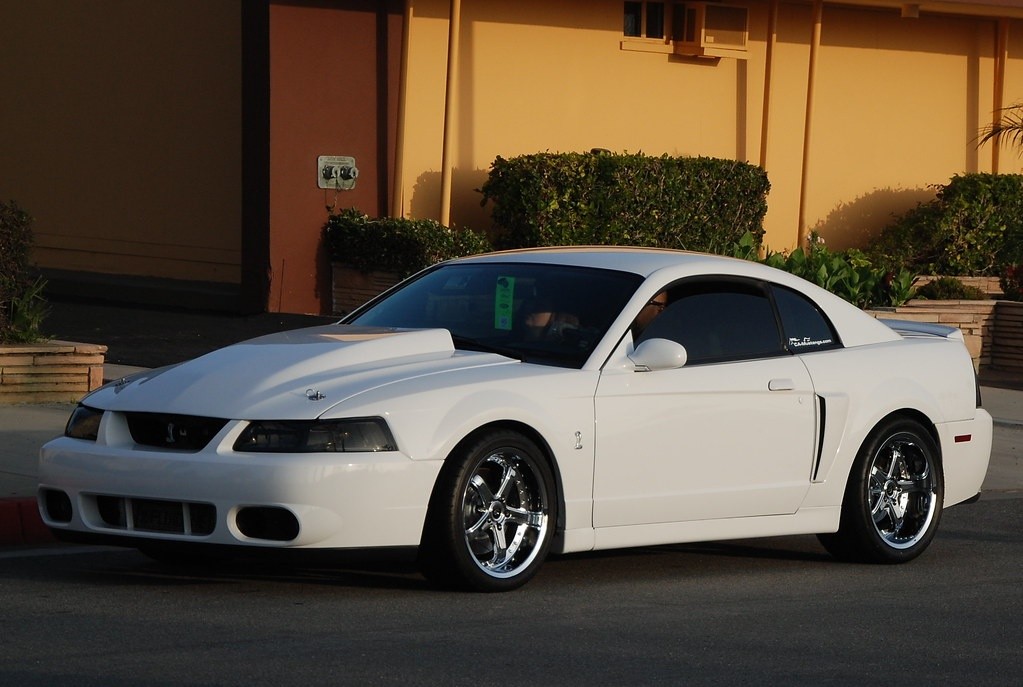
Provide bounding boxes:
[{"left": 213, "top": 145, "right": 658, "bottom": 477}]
[{"left": 35, "top": 244, "right": 994, "bottom": 595}]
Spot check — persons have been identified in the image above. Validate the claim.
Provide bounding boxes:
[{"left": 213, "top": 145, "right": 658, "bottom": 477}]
[{"left": 553, "top": 290, "right": 710, "bottom": 362}]
